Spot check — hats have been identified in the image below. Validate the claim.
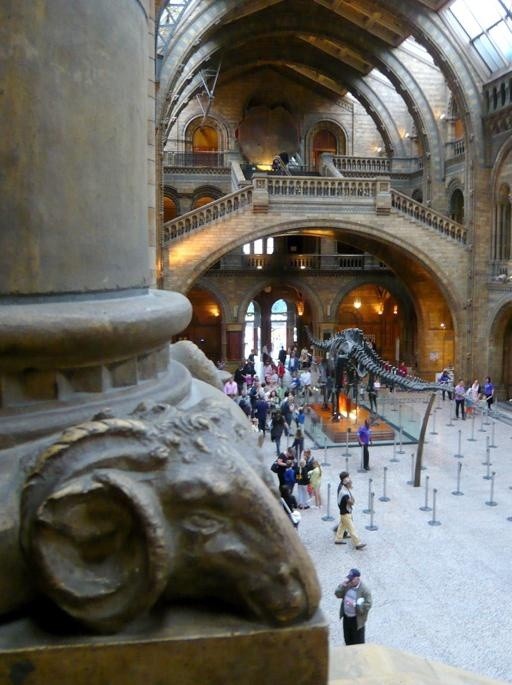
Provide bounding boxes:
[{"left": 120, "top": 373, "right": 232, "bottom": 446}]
[{"left": 346, "top": 568, "right": 360, "bottom": 580}]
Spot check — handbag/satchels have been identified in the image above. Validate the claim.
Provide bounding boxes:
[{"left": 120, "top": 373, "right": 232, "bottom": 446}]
[
  {"left": 289, "top": 508, "right": 301, "bottom": 524},
  {"left": 271, "top": 461, "right": 286, "bottom": 473}
]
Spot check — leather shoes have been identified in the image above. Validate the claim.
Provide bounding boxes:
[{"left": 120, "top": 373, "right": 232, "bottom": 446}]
[
  {"left": 334, "top": 540, "right": 347, "bottom": 544},
  {"left": 356, "top": 543, "right": 366, "bottom": 549}
]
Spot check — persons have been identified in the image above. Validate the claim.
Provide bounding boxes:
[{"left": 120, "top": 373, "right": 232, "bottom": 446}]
[
  {"left": 483, "top": 375, "right": 495, "bottom": 412},
  {"left": 356, "top": 419, "right": 373, "bottom": 472},
  {"left": 331, "top": 471, "right": 352, "bottom": 539},
  {"left": 333, "top": 477, "right": 366, "bottom": 550},
  {"left": 333, "top": 568, "right": 372, "bottom": 646},
  {"left": 437, "top": 370, "right": 452, "bottom": 401},
  {"left": 454, "top": 379, "right": 466, "bottom": 421},
  {"left": 272, "top": 155, "right": 281, "bottom": 173},
  {"left": 396, "top": 360, "right": 409, "bottom": 392},
  {"left": 470, "top": 378, "right": 480, "bottom": 417},
  {"left": 221, "top": 343, "right": 323, "bottom": 532}
]
